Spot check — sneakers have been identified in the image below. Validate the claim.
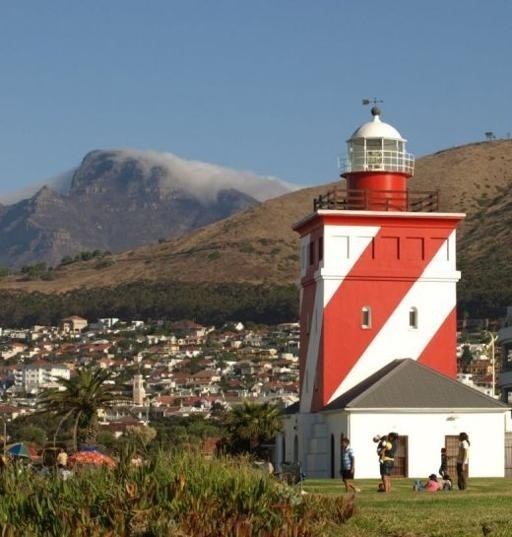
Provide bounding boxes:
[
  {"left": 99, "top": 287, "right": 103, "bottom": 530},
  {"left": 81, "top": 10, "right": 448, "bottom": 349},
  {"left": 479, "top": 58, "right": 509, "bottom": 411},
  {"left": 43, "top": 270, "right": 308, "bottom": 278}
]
[{"left": 354, "top": 487, "right": 361, "bottom": 494}]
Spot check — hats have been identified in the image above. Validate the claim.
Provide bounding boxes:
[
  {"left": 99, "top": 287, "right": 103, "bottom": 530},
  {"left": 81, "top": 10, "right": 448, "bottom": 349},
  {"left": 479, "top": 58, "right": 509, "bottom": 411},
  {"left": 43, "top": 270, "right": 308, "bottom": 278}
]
[{"left": 429, "top": 474, "right": 436, "bottom": 479}]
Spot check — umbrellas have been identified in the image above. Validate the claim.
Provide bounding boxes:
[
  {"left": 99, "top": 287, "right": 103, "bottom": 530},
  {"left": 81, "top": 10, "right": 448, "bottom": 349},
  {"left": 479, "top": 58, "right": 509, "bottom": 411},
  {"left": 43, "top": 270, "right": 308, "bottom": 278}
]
[{"left": 3, "top": 443, "right": 36, "bottom": 458}]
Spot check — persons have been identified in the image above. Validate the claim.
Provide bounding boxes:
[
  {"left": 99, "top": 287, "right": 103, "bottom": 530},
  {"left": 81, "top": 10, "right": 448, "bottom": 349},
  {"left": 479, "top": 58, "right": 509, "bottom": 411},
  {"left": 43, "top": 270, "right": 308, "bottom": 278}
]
[
  {"left": 340, "top": 437, "right": 361, "bottom": 497},
  {"left": 379, "top": 432, "right": 396, "bottom": 494},
  {"left": 376, "top": 436, "right": 387, "bottom": 463},
  {"left": 68, "top": 455, "right": 76, "bottom": 464},
  {"left": 455, "top": 432, "right": 470, "bottom": 491},
  {"left": 413, "top": 448, "right": 452, "bottom": 492},
  {"left": 56, "top": 446, "right": 68, "bottom": 468}
]
